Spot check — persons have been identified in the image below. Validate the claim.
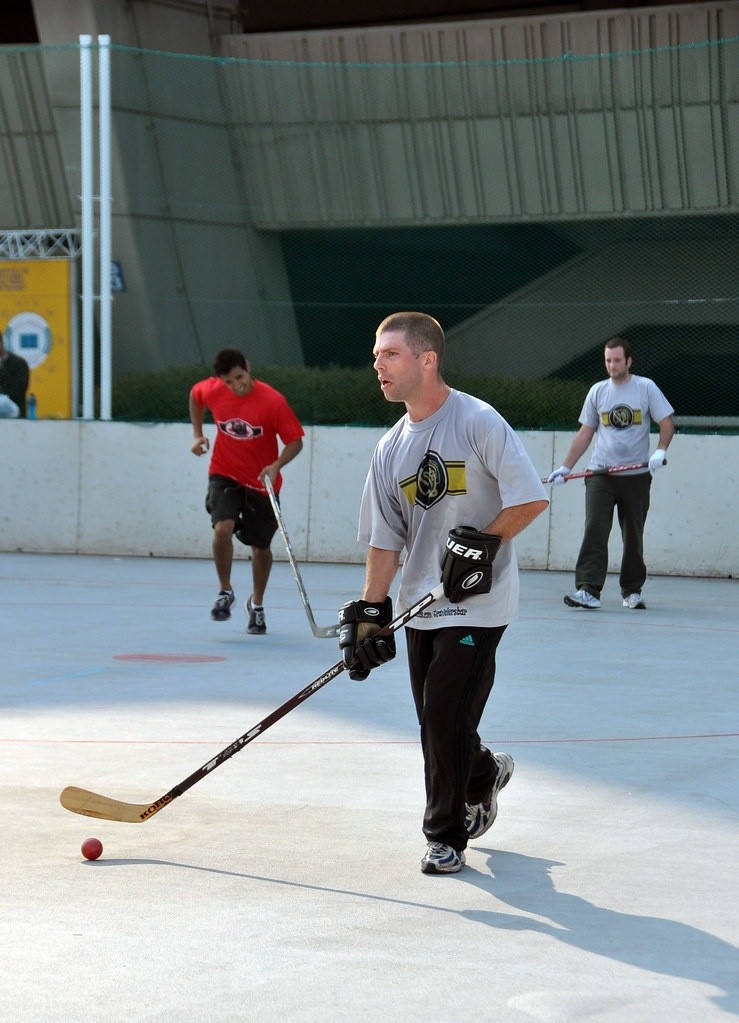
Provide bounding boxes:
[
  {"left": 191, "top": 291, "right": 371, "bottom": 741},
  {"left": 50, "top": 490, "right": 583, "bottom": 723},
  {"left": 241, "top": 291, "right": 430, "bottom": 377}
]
[
  {"left": 336, "top": 310, "right": 548, "bottom": 877},
  {"left": 188, "top": 348, "right": 305, "bottom": 634},
  {"left": 541, "top": 337, "right": 676, "bottom": 609},
  {"left": 0, "top": 332, "right": 32, "bottom": 418}
]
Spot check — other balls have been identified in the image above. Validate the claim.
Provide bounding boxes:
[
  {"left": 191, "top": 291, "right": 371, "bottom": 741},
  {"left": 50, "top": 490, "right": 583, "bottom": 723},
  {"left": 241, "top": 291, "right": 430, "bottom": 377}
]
[{"left": 80, "top": 838, "right": 104, "bottom": 861}]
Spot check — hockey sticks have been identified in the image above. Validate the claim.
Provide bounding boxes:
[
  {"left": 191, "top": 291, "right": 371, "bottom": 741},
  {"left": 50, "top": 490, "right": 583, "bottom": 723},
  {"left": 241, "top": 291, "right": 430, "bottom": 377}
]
[
  {"left": 540, "top": 458, "right": 668, "bottom": 484},
  {"left": 261, "top": 466, "right": 341, "bottom": 639},
  {"left": 57, "top": 578, "right": 447, "bottom": 825}
]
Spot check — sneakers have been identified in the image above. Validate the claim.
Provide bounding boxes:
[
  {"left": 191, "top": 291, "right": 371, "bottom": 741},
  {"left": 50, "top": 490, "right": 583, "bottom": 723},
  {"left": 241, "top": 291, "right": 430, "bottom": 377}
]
[
  {"left": 564, "top": 590, "right": 601, "bottom": 608},
  {"left": 464, "top": 752, "right": 515, "bottom": 839},
  {"left": 246, "top": 594, "right": 266, "bottom": 634},
  {"left": 210, "top": 589, "right": 235, "bottom": 621},
  {"left": 420, "top": 841, "right": 466, "bottom": 874},
  {"left": 623, "top": 592, "right": 646, "bottom": 609}
]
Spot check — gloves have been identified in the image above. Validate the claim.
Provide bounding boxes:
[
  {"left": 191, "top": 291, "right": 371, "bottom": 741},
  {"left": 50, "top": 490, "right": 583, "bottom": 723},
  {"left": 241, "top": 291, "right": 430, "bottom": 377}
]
[
  {"left": 546, "top": 466, "right": 570, "bottom": 486},
  {"left": 338, "top": 596, "right": 396, "bottom": 680},
  {"left": 648, "top": 449, "right": 666, "bottom": 475},
  {"left": 440, "top": 525, "right": 503, "bottom": 603}
]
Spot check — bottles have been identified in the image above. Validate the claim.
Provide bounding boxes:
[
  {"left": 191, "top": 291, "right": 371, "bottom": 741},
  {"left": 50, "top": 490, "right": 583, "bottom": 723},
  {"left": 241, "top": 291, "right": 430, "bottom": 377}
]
[{"left": 27, "top": 392, "right": 37, "bottom": 419}]
